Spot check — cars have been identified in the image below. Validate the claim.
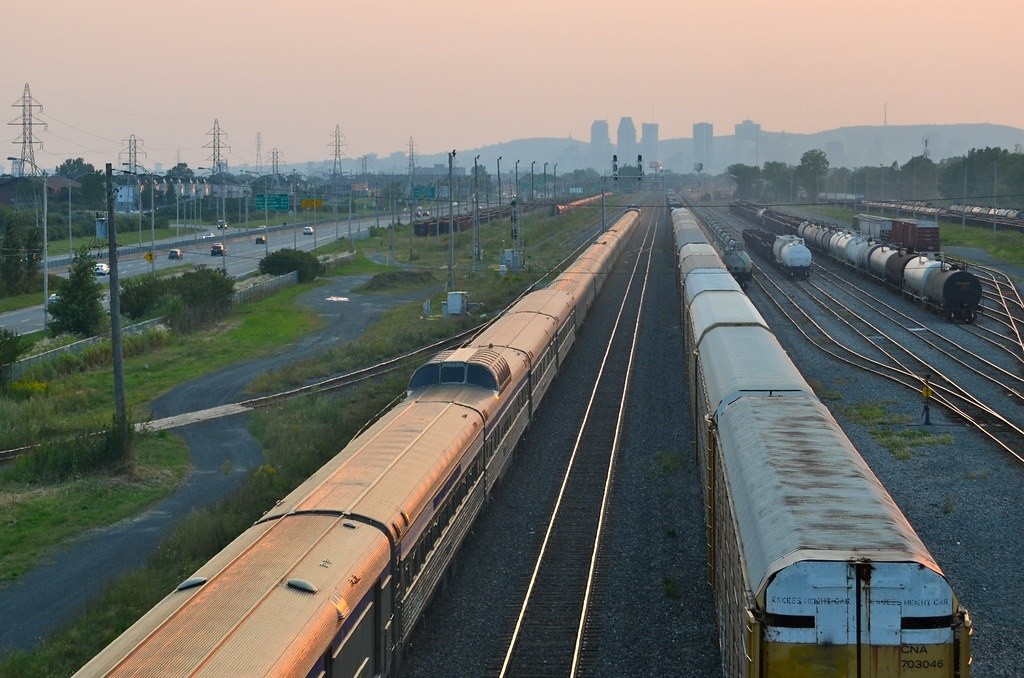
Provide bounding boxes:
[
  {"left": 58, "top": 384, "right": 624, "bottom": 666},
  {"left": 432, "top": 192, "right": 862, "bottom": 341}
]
[{"left": 49, "top": 206, "right": 431, "bottom": 305}]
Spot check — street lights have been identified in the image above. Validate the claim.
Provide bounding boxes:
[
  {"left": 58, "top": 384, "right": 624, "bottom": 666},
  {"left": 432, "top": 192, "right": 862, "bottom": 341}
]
[
  {"left": 122, "top": 162, "right": 156, "bottom": 277},
  {"left": 497, "top": 156, "right": 506, "bottom": 273},
  {"left": 543, "top": 162, "right": 548, "bottom": 207},
  {"left": 554, "top": 163, "right": 559, "bottom": 218},
  {"left": 69, "top": 171, "right": 97, "bottom": 266},
  {"left": 7, "top": 156, "right": 49, "bottom": 331},
  {"left": 447, "top": 149, "right": 457, "bottom": 291},
  {"left": 472, "top": 154, "right": 483, "bottom": 260},
  {"left": 197, "top": 166, "right": 227, "bottom": 276},
  {"left": 531, "top": 160, "right": 535, "bottom": 202},
  {"left": 514, "top": 159, "right": 520, "bottom": 209}
]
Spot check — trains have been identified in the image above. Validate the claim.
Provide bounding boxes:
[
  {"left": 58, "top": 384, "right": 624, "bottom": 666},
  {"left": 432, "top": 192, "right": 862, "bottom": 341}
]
[
  {"left": 742, "top": 226, "right": 812, "bottom": 279},
  {"left": 666, "top": 185, "right": 976, "bottom": 678},
  {"left": 729, "top": 199, "right": 983, "bottom": 324},
  {"left": 851, "top": 212, "right": 941, "bottom": 256},
  {"left": 71, "top": 209, "right": 644, "bottom": 678}
]
